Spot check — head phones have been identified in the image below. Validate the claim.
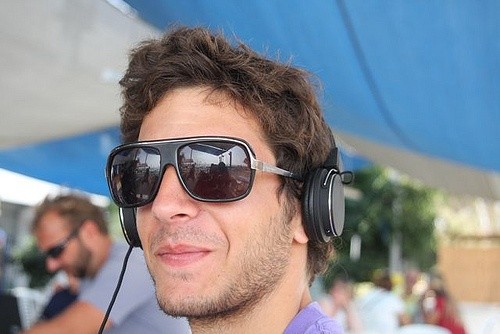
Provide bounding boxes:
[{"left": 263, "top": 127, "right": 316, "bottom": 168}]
[{"left": 118, "top": 130, "right": 346, "bottom": 249}]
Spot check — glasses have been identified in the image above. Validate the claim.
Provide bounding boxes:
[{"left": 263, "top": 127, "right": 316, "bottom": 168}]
[
  {"left": 105, "top": 137, "right": 303, "bottom": 208},
  {"left": 41, "top": 220, "right": 84, "bottom": 259}
]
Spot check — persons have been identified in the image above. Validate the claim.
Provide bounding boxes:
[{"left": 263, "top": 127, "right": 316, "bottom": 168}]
[
  {"left": 316, "top": 267, "right": 471, "bottom": 334},
  {"left": 190, "top": 161, "right": 241, "bottom": 200},
  {"left": 104, "top": 19, "right": 355, "bottom": 334},
  {"left": 22, "top": 192, "right": 193, "bottom": 333},
  {"left": 112, "top": 160, "right": 150, "bottom": 203}
]
